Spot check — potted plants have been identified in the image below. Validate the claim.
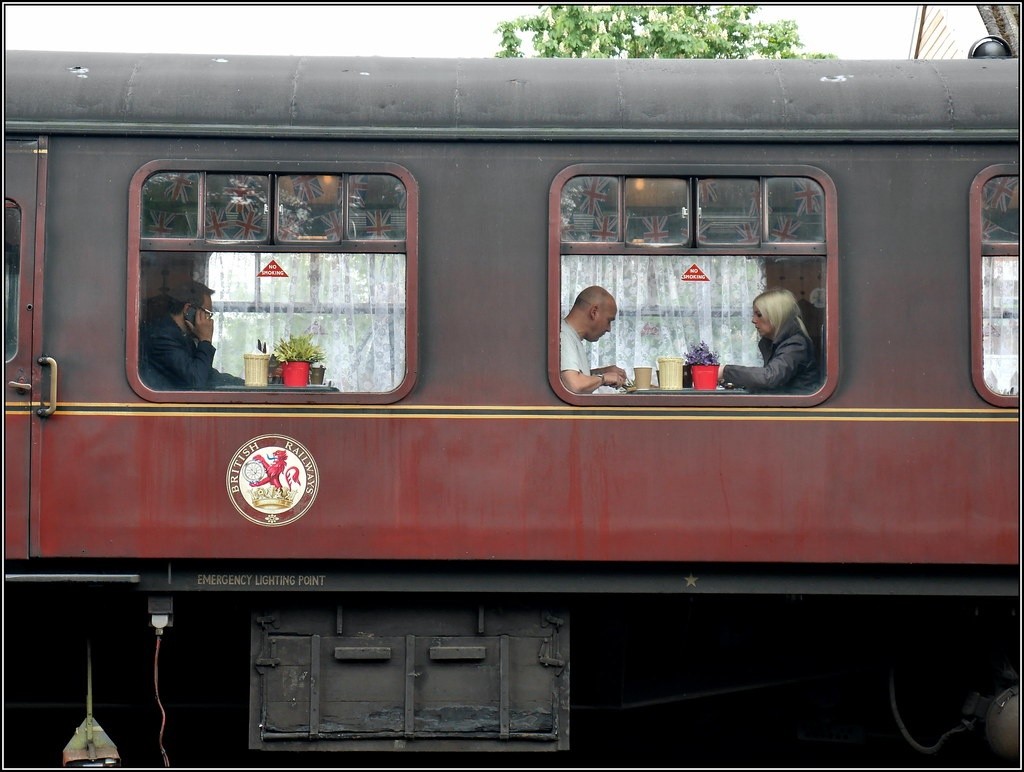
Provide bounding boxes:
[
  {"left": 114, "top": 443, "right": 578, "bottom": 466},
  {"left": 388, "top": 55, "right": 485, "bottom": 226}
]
[{"left": 273, "top": 333, "right": 328, "bottom": 387}]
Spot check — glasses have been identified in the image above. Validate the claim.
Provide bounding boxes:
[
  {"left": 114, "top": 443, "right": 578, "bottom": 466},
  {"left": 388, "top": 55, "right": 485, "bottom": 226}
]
[{"left": 194, "top": 301, "right": 214, "bottom": 320}]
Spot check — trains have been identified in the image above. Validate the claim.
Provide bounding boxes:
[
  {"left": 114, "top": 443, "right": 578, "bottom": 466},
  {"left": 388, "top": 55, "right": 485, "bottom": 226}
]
[{"left": 1, "top": 37, "right": 1018, "bottom": 767}]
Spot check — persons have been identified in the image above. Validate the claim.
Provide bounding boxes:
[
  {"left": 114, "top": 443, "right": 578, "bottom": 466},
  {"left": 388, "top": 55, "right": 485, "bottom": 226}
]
[
  {"left": 717, "top": 287, "right": 818, "bottom": 389},
  {"left": 142, "top": 282, "right": 247, "bottom": 391},
  {"left": 560, "top": 286, "right": 627, "bottom": 395}
]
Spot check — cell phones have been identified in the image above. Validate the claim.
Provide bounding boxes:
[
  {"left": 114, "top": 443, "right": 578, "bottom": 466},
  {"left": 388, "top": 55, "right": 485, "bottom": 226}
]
[{"left": 184, "top": 307, "right": 196, "bottom": 327}]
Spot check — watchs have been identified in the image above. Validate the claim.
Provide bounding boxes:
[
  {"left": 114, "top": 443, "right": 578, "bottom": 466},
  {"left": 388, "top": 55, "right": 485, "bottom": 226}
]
[{"left": 597, "top": 375, "right": 604, "bottom": 383}]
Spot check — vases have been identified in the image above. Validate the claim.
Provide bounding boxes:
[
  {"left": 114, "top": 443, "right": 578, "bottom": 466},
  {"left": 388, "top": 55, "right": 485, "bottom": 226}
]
[{"left": 690, "top": 365, "right": 719, "bottom": 390}]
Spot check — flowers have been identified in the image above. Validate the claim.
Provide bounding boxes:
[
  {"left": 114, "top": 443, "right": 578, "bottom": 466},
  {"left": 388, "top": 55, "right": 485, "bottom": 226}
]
[{"left": 683, "top": 340, "right": 721, "bottom": 367}]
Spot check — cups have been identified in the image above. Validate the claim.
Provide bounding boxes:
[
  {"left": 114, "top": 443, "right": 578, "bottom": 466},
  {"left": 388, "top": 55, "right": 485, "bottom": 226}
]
[
  {"left": 683, "top": 366, "right": 692, "bottom": 388},
  {"left": 658, "top": 357, "right": 684, "bottom": 389},
  {"left": 243, "top": 353, "right": 270, "bottom": 388},
  {"left": 635, "top": 367, "right": 651, "bottom": 391},
  {"left": 309, "top": 365, "right": 325, "bottom": 385}
]
